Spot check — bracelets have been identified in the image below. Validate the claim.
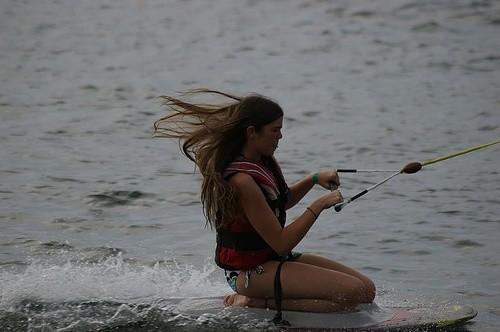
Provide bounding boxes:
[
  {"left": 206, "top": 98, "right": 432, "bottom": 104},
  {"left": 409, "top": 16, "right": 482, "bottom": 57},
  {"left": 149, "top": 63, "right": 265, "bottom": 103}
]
[
  {"left": 313, "top": 171, "right": 319, "bottom": 184},
  {"left": 307, "top": 206, "right": 318, "bottom": 219}
]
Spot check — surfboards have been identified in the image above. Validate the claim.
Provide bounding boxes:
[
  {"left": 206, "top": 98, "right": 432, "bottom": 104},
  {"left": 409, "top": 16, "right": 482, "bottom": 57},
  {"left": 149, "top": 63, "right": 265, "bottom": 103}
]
[{"left": 163, "top": 296, "right": 482, "bottom": 330}]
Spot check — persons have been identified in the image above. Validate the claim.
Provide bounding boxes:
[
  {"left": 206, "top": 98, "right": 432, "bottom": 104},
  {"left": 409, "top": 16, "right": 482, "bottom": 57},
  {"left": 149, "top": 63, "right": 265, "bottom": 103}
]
[{"left": 151, "top": 85, "right": 377, "bottom": 312}]
